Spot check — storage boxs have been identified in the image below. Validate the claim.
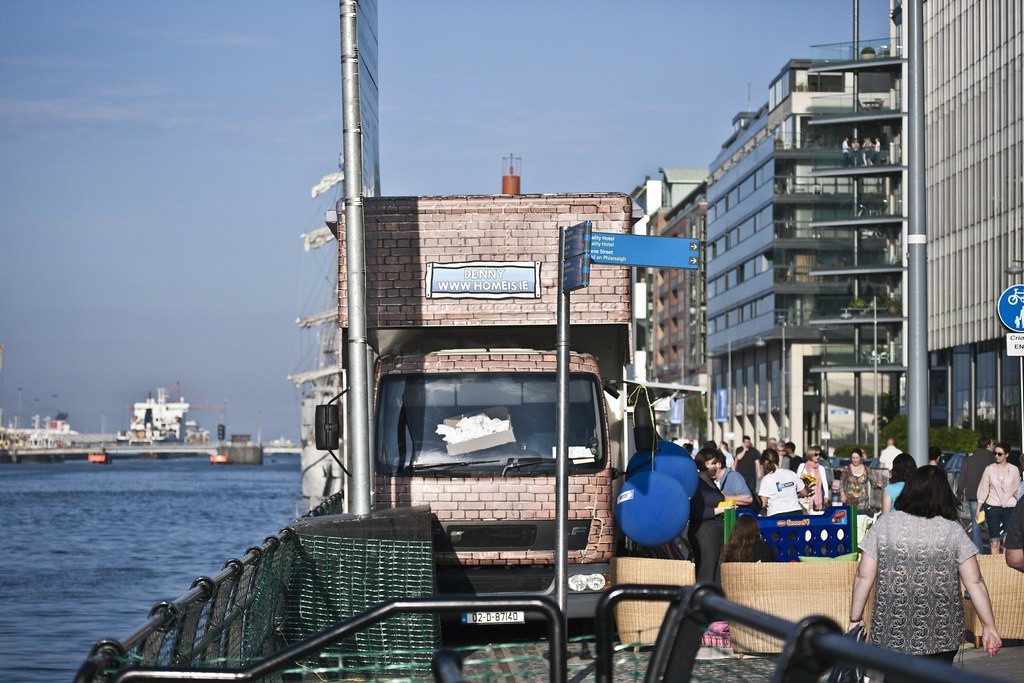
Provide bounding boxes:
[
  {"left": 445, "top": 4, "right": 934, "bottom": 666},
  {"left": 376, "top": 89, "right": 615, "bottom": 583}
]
[{"left": 442, "top": 405, "right": 516, "bottom": 456}]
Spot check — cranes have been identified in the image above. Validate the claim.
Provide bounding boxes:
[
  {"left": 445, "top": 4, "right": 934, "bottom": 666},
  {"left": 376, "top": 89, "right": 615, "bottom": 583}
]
[{"left": 165, "top": 382, "right": 227, "bottom": 411}]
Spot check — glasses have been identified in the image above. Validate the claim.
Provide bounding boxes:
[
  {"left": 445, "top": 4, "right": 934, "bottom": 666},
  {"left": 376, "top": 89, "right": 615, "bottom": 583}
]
[
  {"left": 993, "top": 450, "right": 1007, "bottom": 456},
  {"left": 814, "top": 453, "right": 821, "bottom": 457}
]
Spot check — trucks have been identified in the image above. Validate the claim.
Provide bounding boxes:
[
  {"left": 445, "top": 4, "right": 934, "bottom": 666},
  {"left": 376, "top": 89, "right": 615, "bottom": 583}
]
[{"left": 314, "top": 193, "right": 632, "bottom": 628}]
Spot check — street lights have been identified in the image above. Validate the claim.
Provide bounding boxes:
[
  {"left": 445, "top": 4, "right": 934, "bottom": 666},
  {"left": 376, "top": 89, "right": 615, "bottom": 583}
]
[
  {"left": 841, "top": 307, "right": 879, "bottom": 461},
  {"left": 755, "top": 336, "right": 786, "bottom": 440},
  {"left": 704, "top": 350, "right": 731, "bottom": 451}
]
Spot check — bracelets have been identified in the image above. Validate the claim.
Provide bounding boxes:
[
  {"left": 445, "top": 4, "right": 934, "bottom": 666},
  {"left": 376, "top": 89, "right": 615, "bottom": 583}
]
[{"left": 849, "top": 617, "right": 862, "bottom": 623}]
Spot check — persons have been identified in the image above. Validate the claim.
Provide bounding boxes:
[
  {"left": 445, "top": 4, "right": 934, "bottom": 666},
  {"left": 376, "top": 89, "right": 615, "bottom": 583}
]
[
  {"left": 848, "top": 465, "right": 1002, "bottom": 683},
  {"left": 796, "top": 445, "right": 829, "bottom": 512},
  {"left": 757, "top": 448, "right": 808, "bottom": 516},
  {"left": 671, "top": 435, "right": 1024, "bottom": 574},
  {"left": 686, "top": 448, "right": 739, "bottom": 620},
  {"left": 841, "top": 136, "right": 884, "bottom": 168},
  {"left": 976, "top": 441, "right": 1021, "bottom": 555},
  {"left": 720, "top": 514, "right": 778, "bottom": 562}
]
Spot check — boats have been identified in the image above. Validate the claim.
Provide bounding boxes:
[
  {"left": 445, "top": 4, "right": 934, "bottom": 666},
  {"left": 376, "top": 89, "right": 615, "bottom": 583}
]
[
  {"left": 115, "top": 386, "right": 191, "bottom": 446},
  {"left": 25, "top": 431, "right": 62, "bottom": 449}
]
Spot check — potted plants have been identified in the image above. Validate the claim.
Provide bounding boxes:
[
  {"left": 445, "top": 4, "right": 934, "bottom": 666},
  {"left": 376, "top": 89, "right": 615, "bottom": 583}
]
[{"left": 886, "top": 302, "right": 899, "bottom": 316}]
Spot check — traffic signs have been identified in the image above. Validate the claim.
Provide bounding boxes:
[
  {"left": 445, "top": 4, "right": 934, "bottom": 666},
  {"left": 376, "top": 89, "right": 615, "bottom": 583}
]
[{"left": 563, "top": 218, "right": 701, "bottom": 294}]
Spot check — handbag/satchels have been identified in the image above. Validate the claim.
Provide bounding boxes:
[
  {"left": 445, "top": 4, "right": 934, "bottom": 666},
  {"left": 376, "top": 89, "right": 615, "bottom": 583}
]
[
  {"left": 826, "top": 623, "right": 868, "bottom": 683},
  {"left": 735, "top": 493, "right": 762, "bottom": 515}
]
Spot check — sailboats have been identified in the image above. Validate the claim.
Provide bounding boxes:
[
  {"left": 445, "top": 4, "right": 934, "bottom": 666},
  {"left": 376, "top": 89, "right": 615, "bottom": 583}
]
[{"left": 290, "top": 167, "right": 345, "bottom": 506}]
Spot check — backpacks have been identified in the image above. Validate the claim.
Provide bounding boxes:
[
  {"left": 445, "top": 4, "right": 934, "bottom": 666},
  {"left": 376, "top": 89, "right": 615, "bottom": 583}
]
[{"left": 783, "top": 454, "right": 805, "bottom": 473}]
